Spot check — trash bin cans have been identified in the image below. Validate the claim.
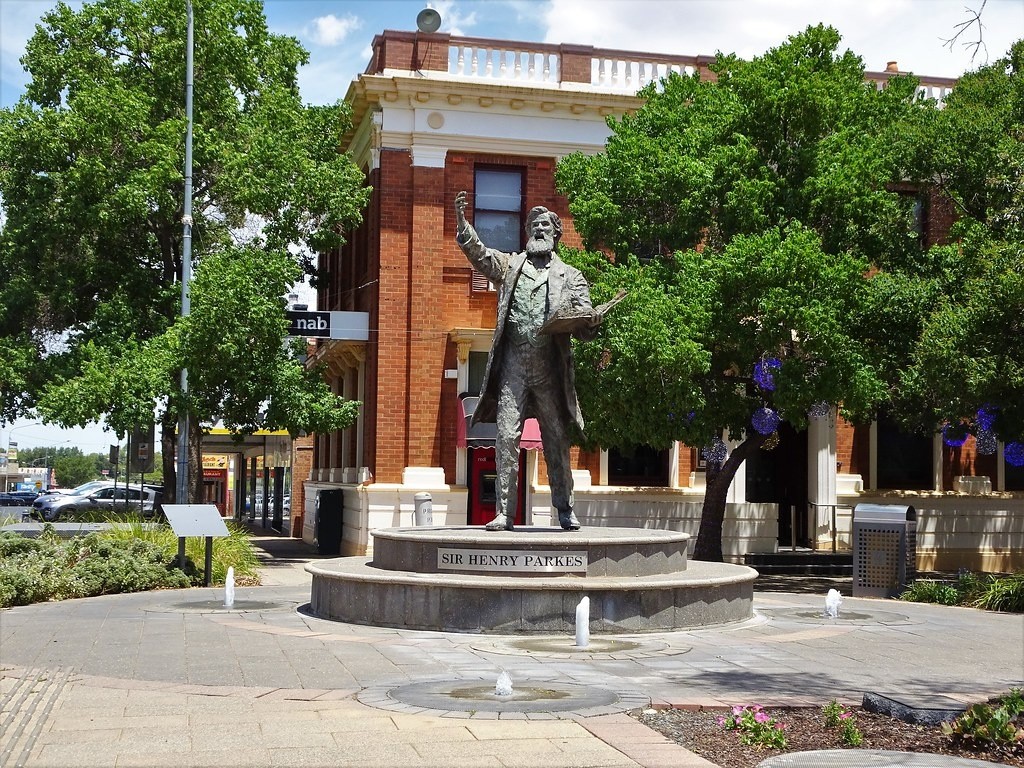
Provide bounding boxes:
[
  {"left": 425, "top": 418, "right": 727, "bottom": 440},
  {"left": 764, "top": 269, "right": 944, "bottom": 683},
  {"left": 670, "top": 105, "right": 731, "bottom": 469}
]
[{"left": 852, "top": 503, "right": 917, "bottom": 599}]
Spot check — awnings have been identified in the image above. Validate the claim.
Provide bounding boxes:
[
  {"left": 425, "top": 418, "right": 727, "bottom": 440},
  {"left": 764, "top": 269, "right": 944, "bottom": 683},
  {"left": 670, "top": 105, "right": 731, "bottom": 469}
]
[{"left": 456, "top": 392, "right": 543, "bottom": 450}]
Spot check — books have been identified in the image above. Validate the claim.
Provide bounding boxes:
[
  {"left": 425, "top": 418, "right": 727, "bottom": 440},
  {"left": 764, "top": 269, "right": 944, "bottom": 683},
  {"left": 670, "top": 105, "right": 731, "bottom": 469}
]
[{"left": 535, "top": 290, "right": 628, "bottom": 336}]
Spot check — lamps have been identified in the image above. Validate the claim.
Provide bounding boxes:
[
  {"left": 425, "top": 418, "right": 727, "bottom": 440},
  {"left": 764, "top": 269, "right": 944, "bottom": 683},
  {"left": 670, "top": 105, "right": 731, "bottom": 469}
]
[{"left": 414, "top": 3, "right": 441, "bottom": 67}]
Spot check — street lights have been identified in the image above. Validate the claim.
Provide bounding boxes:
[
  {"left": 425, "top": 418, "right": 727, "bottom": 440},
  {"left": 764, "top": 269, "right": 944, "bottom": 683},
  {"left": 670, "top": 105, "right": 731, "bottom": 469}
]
[
  {"left": 5, "top": 422, "right": 41, "bottom": 494},
  {"left": 45, "top": 440, "right": 71, "bottom": 491}
]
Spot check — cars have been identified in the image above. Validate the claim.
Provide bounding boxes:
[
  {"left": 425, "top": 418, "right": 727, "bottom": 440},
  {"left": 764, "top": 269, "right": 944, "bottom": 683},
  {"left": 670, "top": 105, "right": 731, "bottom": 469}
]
[
  {"left": 246, "top": 493, "right": 290, "bottom": 517},
  {"left": 31, "top": 480, "right": 163, "bottom": 523},
  {"left": 0, "top": 490, "right": 39, "bottom": 507}
]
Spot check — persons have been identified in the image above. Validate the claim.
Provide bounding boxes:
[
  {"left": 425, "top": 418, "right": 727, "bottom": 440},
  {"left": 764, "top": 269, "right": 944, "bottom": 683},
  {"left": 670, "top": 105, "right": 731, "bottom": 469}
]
[{"left": 454, "top": 190, "right": 604, "bottom": 532}]
